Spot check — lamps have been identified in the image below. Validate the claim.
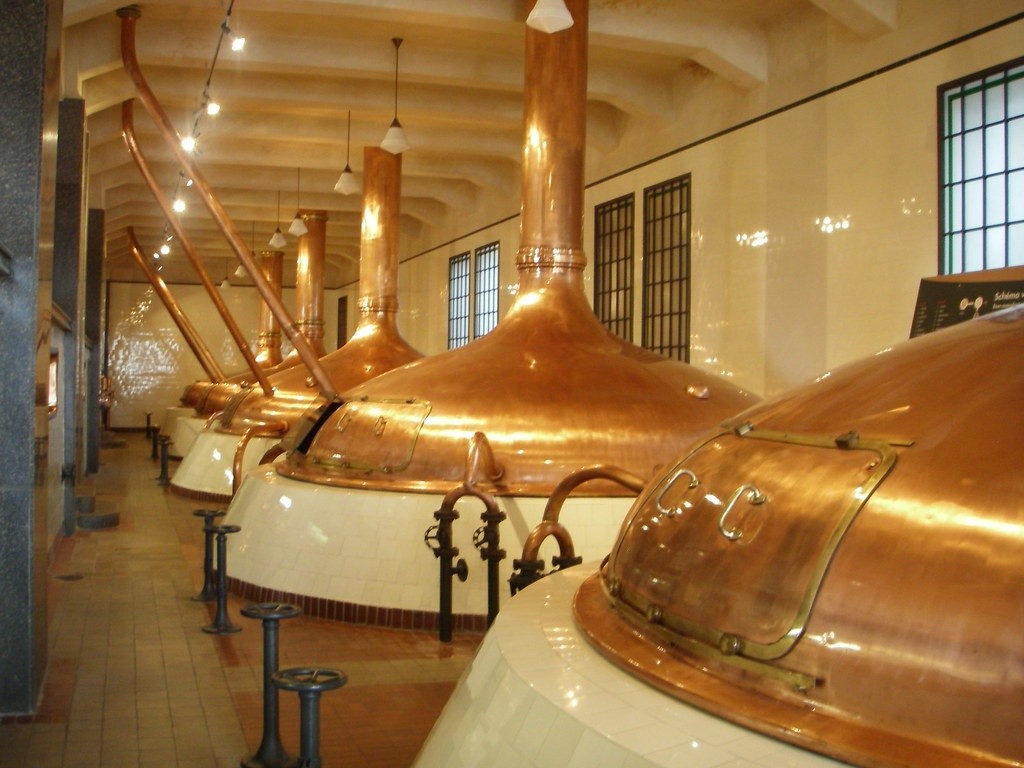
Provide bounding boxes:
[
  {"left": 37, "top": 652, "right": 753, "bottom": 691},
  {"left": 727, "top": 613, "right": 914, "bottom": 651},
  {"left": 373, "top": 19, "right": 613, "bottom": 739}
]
[
  {"left": 235, "top": 263, "right": 249, "bottom": 279},
  {"left": 380, "top": 37, "right": 412, "bottom": 155},
  {"left": 332, "top": 110, "right": 362, "bottom": 196},
  {"left": 526, "top": 0, "right": 574, "bottom": 35},
  {"left": 288, "top": 168, "right": 307, "bottom": 236},
  {"left": 221, "top": 258, "right": 232, "bottom": 290},
  {"left": 269, "top": 190, "right": 286, "bottom": 249}
]
